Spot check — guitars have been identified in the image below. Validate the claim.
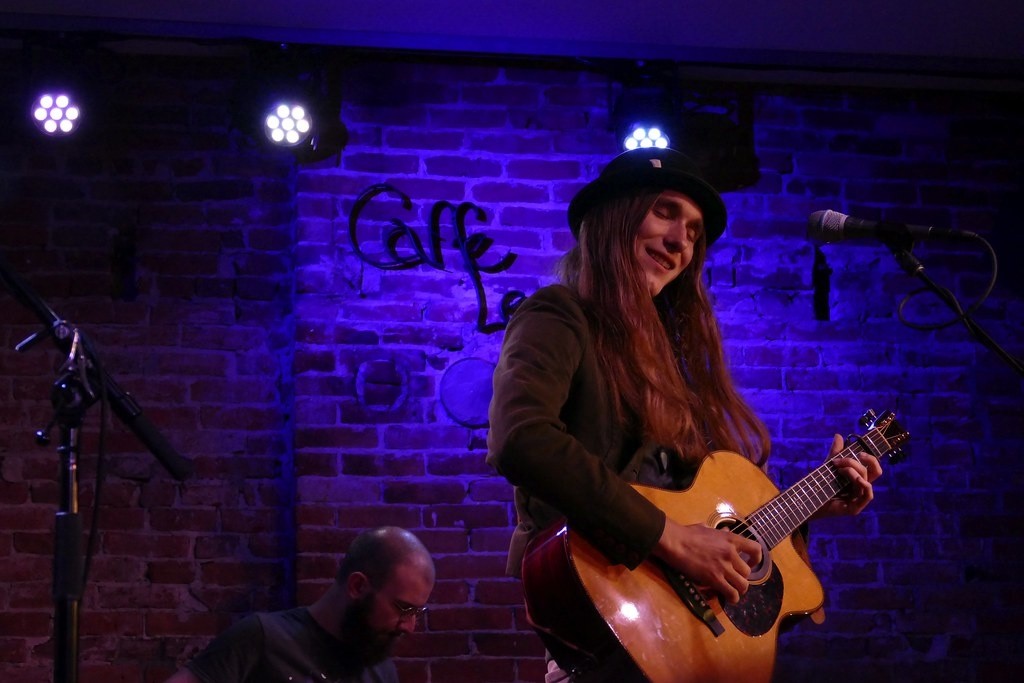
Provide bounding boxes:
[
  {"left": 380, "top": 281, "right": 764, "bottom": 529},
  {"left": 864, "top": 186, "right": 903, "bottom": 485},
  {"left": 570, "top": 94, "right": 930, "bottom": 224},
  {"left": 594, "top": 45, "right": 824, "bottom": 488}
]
[{"left": 519, "top": 407, "right": 913, "bottom": 683}]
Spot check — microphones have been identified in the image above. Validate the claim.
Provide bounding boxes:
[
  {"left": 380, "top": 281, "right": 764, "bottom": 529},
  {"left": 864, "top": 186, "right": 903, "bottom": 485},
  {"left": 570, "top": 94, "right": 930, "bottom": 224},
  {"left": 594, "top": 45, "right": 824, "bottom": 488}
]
[{"left": 807, "top": 209, "right": 980, "bottom": 243}]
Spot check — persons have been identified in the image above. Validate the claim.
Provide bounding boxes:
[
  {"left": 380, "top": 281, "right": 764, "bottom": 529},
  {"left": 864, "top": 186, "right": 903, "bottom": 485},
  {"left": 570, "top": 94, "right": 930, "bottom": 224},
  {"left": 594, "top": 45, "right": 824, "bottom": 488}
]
[
  {"left": 483, "top": 148, "right": 883, "bottom": 683},
  {"left": 168, "top": 526, "right": 437, "bottom": 683}
]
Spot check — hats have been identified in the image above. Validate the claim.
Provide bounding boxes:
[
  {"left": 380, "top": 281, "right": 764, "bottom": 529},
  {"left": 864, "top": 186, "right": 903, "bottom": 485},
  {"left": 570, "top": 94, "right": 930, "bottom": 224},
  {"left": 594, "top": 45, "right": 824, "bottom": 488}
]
[{"left": 568, "top": 147, "right": 727, "bottom": 247}]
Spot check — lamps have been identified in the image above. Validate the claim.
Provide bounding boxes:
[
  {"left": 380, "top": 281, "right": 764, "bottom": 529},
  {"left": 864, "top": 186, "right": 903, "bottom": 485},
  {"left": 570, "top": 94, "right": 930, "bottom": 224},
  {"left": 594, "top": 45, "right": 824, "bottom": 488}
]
[
  {"left": 618, "top": 109, "right": 670, "bottom": 151},
  {"left": 263, "top": 99, "right": 352, "bottom": 167}
]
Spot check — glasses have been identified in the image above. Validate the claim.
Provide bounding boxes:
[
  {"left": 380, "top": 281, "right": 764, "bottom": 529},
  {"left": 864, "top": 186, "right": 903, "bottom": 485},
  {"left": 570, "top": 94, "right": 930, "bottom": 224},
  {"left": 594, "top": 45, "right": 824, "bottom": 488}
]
[{"left": 378, "top": 589, "right": 429, "bottom": 619}]
